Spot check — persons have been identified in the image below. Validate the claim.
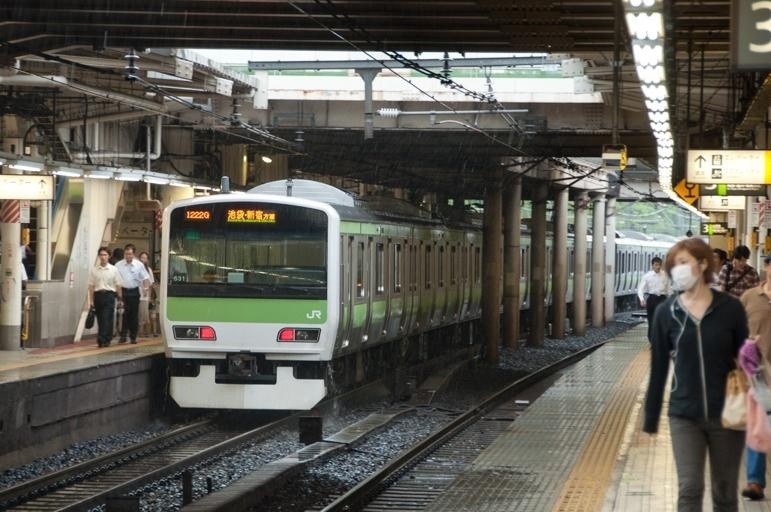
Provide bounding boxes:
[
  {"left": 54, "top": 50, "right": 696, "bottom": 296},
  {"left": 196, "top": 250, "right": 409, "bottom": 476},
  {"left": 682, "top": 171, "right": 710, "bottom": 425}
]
[
  {"left": 20, "top": 244, "right": 35, "bottom": 291},
  {"left": 88, "top": 243, "right": 156, "bottom": 348},
  {"left": 637, "top": 236, "right": 770, "bottom": 512}
]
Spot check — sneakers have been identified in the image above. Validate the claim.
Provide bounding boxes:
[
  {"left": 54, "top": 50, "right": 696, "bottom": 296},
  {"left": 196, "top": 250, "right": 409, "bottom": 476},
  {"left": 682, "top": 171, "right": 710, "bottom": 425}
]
[{"left": 741, "top": 483, "right": 764, "bottom": 500}]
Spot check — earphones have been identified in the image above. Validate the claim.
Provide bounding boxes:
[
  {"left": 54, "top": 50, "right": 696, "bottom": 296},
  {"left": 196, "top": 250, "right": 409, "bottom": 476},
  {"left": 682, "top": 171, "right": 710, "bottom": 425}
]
[{"left": 701, "top": 263, "right": 705, "bottom": 272}]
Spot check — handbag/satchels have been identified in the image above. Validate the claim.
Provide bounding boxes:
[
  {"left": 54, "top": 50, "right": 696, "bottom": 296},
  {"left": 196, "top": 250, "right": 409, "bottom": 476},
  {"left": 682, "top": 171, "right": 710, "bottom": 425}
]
[
  {"left": 86, "top": 311, "right": 94, "bottom": 329},
  {"left": 720, "top": 370, "right": 750, "bottom": 432}
]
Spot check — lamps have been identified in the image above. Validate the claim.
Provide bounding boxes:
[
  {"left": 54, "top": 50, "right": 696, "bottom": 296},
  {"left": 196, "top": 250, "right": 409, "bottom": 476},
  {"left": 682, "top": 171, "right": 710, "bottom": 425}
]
[
  {"left": 621, "top": 0, "right": 710, "bottom": 224},
  {"left": 0, "top": 151, "right": 242, "bottom": 195},
  {"left": 261, "top": 148, "right": 275, "bottom": 163}
]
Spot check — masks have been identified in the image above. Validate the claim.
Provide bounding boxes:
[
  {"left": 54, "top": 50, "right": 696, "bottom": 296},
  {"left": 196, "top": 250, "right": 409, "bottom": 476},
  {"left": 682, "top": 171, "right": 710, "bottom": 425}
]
[{"left": 670, "top": 259, "right": 702, "bottom": 291}]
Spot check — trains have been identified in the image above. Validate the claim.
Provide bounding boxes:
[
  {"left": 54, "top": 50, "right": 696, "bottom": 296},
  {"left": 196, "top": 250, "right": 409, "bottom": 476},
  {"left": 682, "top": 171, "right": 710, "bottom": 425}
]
[{"left": 160, "top": 178, "right": 676, "bottom": 417}]
